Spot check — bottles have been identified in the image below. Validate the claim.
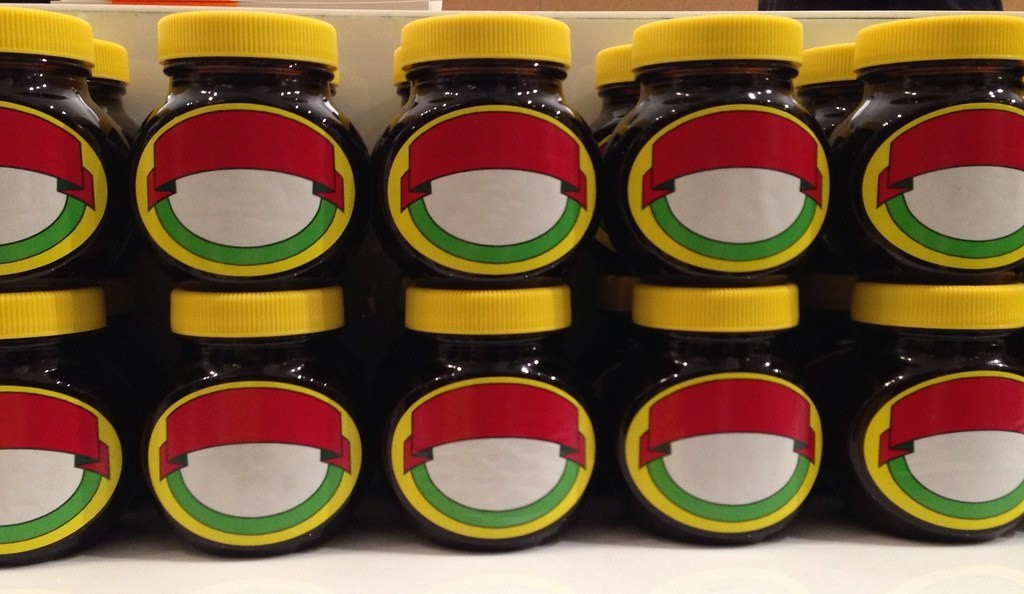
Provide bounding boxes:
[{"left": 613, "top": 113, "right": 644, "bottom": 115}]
[
  {"left": 369, "top": 14, "right": 606, "bottom": 287},
  {"left": 587, "top": 15, "right": 842, "bottom": 288},
  {"left": 137, "top": 290, "right": 380, "bottom": 560},
  {"left": 0, "top": 290, "right": 139, "bottom": 569},
  {"left": 611, "top": 285, "right": 842, "bottom": 547},
  {"left": 379, "top": 286, "right": 611, "bottom": 555},
  {"left": 135, "top": 10, "right": 370, "bottom": 289},
  {"left": 0, "top": 5, "right": 138, "bottom": 292},
  {"left": 843, "top": 283, "right": 1024, "bottom": 544},
  {"left": 797, "top": 15, "right": 1024, "bottom": 285}
]
